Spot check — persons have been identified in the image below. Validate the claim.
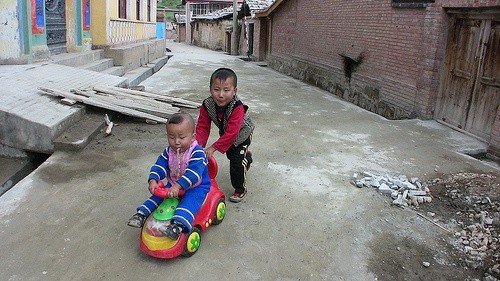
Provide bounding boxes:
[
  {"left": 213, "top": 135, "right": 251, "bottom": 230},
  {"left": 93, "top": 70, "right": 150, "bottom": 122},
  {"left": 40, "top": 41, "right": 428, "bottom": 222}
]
[
  {"left": 194, "top": 67, "right": 256, "bottom": 203},
  {"left": 127, "top": 111, "right": 211, "bottom": 240}
]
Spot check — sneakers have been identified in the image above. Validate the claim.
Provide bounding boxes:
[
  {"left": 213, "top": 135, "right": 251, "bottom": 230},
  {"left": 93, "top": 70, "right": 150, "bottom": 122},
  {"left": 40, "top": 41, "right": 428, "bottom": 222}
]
[
  {"left": 230, "top": 193, "right": 246, "bottom": 202},
  {"left": 247, "top": 151, "right": 252, "bottom": 169}
]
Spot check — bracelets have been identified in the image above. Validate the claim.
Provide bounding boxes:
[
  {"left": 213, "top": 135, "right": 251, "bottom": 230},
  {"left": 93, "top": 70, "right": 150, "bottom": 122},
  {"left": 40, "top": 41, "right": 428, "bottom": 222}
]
[{"left": 149, "top": 179, "right": 156, "bottom": 184}]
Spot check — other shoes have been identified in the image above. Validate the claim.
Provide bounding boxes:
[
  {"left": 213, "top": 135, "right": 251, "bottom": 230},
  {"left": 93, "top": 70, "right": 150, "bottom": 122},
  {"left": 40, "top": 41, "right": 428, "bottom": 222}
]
[
  {"left": 159, "top": 224, "right": 180, "bottom": 241},
  {"left": 127, "top": 213, "right": 146, "bottom": 228}
]
